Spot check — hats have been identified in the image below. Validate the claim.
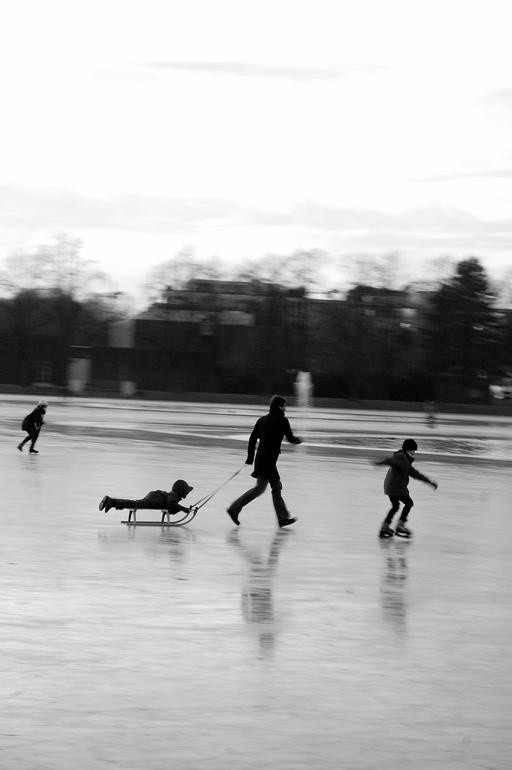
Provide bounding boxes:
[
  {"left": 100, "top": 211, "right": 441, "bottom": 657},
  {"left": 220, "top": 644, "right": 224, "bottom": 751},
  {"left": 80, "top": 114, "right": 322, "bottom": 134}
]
[
  {"left": 171, "top": 478, "right": 193, "bottom": 498},
  {"left": 401, "top": 438, "right": 418, "bottom": 452},
  {"left": 36, "top": 398, "right": 48, "bottom": 407}
]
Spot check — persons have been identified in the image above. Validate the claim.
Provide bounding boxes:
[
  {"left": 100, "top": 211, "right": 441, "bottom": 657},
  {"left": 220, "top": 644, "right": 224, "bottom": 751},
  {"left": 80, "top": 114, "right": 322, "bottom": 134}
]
[
  {"left": 17, "top": 399, "right": 49, "bottom": 453},
  {"left": 375, "top": 536, "right": 413, "bottom": 651},
  {"left": 371, "top": 438, "right": 439, "bottom": 536},
  {"left": 98, "top": 478, "right": 193, "bottom": 514},
  {"left": 225, "top": 528, "right": 295, "bottom": 662},
  {"left": 227, "top": 395, "right": 306, "bottom": 528}
]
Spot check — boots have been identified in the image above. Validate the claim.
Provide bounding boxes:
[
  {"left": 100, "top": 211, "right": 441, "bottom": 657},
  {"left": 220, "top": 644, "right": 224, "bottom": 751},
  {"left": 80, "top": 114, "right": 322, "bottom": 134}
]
[{"left": 381, "top": 517, "right": 410, "bottom": 534}]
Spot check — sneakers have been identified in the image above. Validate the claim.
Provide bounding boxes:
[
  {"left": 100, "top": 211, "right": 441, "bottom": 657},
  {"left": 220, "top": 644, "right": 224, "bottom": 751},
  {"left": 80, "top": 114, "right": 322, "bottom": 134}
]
[
  {"left": 17, "top": 444, "right": 40, "bottom": 453},
  {"left": 98, "top": 495, "right": 115, "bottom": 513},
  {"left": 276, "top": 515, "right": 300, "bottom": 528},
  {"left": 226, "top": 505, "right": 241, "bottom": 526}
]
